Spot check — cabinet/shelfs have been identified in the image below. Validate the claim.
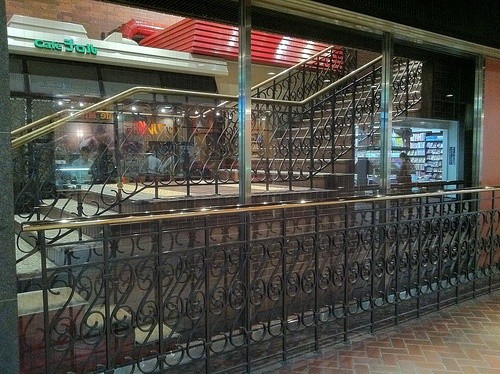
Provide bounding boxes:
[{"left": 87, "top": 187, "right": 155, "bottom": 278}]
[{"left": 355, "top": 129, "right": 444, "bottom": 197}]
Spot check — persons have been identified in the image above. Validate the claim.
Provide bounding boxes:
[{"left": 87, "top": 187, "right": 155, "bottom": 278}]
[
  {"left": 395, "top": 153, "right": 418, "bottom": 191},
  {"left": 68, "top": 139, "right": 195, "bottom": 184},
  {"left": 355, "top": 153, "right": 374, "bottom": 189}
]
